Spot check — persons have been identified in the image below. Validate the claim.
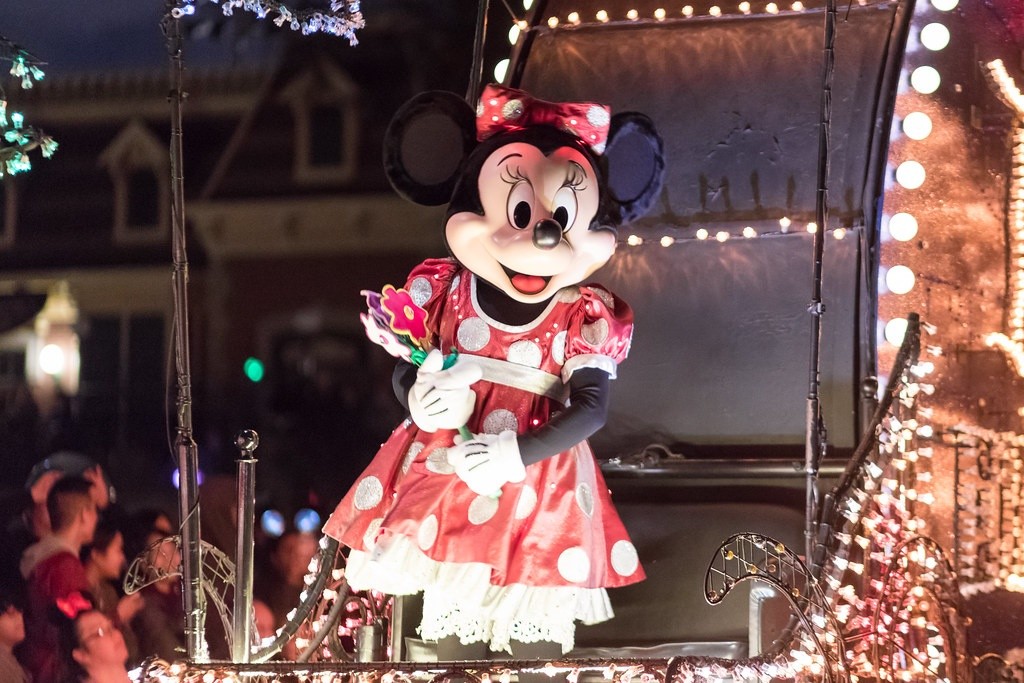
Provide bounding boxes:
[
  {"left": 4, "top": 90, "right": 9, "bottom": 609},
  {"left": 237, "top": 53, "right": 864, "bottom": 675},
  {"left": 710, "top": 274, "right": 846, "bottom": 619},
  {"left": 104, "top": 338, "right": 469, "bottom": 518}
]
[{"left": 0, "top": 451, "right": 317, "bottom": 683}]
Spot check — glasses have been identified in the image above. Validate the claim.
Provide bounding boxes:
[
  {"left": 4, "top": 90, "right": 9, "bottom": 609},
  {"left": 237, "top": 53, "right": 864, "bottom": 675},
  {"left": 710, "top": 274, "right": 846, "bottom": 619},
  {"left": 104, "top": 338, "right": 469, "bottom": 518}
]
[
  {"left": 0, "top": 600, "right": 24, "bottom": 615},
  {"left": 80, "top": 620, "right": 117, "bottom": 644}
]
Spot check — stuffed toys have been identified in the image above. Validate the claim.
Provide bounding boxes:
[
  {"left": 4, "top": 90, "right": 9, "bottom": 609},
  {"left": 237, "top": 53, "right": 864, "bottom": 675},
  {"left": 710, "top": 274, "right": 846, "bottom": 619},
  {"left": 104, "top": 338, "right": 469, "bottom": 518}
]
[{"left": 322, "top": 90, "right": 667, "bottom": 661}]
